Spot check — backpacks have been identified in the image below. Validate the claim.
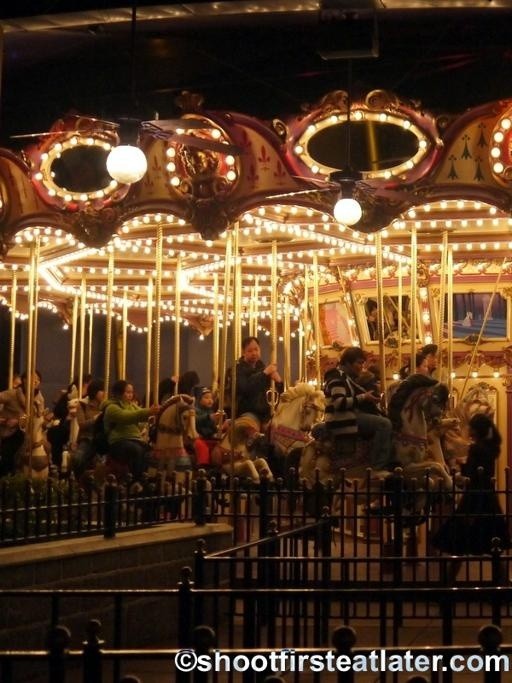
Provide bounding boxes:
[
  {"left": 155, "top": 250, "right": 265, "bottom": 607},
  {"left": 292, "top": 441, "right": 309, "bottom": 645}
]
[{"left": 92, "top": 403, "right": 123, "bottom": 453}]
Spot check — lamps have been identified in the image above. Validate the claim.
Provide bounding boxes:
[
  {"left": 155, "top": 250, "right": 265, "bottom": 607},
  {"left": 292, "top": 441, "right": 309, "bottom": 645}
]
[
  {"left": 331, "top": 195, "right": 365, "bottom": 226},
  {"left": 103, "top": 140, "right": 152, "bottom": 181}
]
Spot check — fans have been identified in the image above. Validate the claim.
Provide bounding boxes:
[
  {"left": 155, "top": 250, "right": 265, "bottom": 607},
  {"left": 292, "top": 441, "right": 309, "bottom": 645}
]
[
  {"left": 10, "top": 1, "right": 243, "bottom": 158},
  {"left": 264, "top": 61, "right": 437, "bottom": 208}
]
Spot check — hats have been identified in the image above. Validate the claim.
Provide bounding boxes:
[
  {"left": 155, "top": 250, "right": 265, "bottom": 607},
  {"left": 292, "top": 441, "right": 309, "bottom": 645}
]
[{"left": 194, "top": 386, "right": 210, "bottom": 399}]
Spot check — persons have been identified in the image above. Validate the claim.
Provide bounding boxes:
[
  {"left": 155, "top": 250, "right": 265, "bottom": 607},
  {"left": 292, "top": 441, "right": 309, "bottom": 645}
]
[
  {"left": 0, "top": 338, "right": 449, "bottom": 481},
  {"left": 430, "top": 413, "right": 512, "bottom": 588}
]
